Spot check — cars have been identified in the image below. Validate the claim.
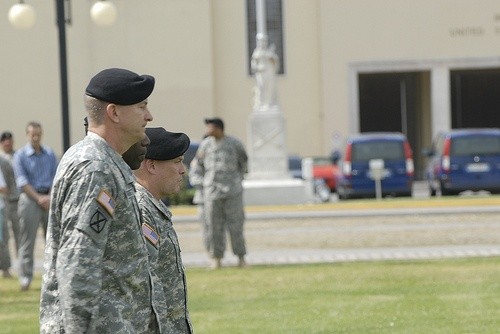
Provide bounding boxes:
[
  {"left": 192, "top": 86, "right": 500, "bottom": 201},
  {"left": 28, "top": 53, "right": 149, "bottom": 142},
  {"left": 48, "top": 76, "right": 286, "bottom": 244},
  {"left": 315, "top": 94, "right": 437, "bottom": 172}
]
[{"left": 287, "top": 156, "right": 339, "bottom": 193}]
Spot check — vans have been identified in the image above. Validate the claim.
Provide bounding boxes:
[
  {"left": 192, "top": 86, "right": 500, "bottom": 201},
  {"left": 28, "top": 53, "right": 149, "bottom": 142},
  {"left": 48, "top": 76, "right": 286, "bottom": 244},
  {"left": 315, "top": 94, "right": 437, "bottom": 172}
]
[
  {"left": 421, "top": 128, "right": 500, "bottom": 197},
  {"left": 335, "top": 132, "right": 415, "bottom": 200}
]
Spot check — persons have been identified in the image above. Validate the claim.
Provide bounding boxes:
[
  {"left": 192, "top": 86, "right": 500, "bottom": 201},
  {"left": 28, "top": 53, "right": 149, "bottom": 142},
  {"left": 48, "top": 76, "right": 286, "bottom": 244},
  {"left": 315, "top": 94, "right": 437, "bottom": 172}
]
[
  {"left": 189, "top": 117, "right": 248, "bottom": 270},
  {"left": 38, "top": 68, "right": 194, "bottom": 334},
  {"left": 0, "top": 121, "right": 59, "bottom": 291},
  {"left": 250, "top": 34, "right": 280, "bottom": 112}
]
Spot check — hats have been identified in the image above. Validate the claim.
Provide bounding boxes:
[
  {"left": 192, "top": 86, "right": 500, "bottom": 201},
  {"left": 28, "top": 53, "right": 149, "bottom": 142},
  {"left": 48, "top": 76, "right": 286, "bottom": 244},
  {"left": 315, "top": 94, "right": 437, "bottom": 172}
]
[
  {"left": 85, "top": 68, "right": 155, "bottom": 106},
  {"left": 145, "top": 128, "right": 190, "bottom": 161},
  {"left": 0, "top": 132, "right": 12, "bottom": 142}
]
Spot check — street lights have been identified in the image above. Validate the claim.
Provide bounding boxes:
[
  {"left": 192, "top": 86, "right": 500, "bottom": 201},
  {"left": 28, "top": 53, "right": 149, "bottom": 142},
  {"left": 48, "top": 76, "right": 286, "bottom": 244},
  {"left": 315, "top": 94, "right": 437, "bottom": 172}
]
[{"left": 7, "top": 0, "right": 118, "bottom": 155}]
